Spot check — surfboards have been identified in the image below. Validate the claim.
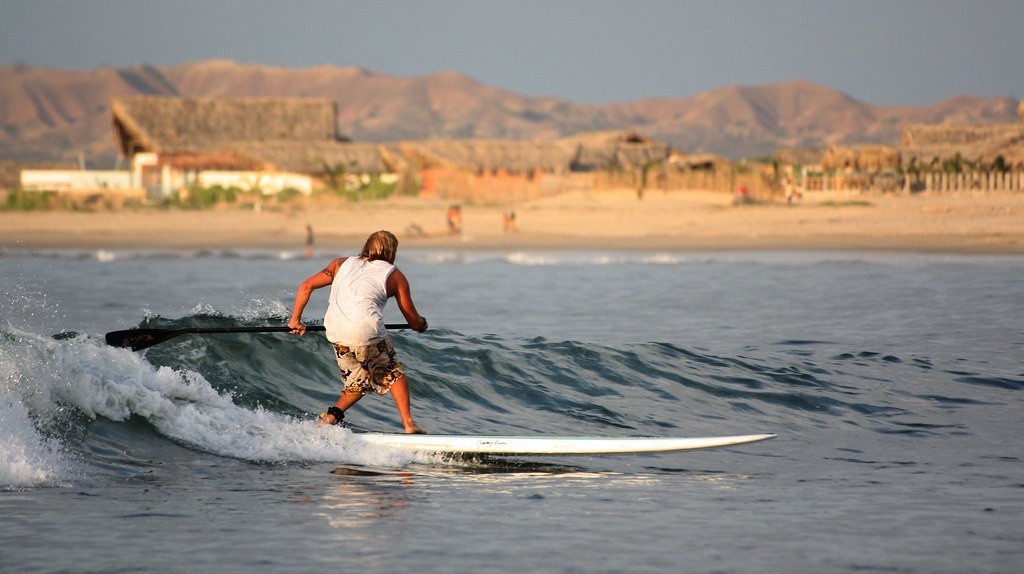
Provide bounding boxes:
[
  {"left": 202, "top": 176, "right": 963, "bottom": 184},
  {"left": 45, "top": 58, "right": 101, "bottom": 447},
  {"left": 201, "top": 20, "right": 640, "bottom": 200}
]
[{"left": 340, "top": 428, "right": 782, "bottom": 458}]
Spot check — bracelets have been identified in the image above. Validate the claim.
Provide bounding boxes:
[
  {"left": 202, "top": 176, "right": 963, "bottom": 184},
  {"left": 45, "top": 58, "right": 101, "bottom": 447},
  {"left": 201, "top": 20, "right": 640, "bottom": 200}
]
[{"left": 420, "top": 316, "right": 426, "bottom": 329}]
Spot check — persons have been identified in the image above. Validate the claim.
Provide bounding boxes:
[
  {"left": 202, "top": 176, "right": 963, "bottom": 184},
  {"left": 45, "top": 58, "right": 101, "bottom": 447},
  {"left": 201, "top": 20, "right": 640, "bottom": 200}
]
[
  {"left": 304, "top": 223, "right": 312, "bottom": 256},
  {"left": 446, "top": 206, "right": 464, "bottom": 235},
  {"left": 288, "top": 230, "right": 430, "bottom": 433},
  {"left": 503, "top": 211, "right": 521, "bottom": 236}
]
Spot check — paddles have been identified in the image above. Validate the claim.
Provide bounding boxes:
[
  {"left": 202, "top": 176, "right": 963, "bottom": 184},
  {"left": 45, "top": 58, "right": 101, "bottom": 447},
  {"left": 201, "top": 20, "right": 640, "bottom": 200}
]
[{"left": 104, "top": 321, "right": 413, "bottom": 355}]
